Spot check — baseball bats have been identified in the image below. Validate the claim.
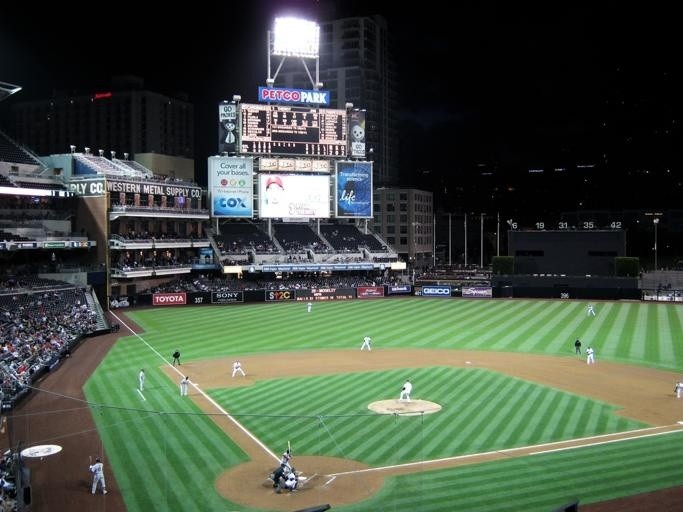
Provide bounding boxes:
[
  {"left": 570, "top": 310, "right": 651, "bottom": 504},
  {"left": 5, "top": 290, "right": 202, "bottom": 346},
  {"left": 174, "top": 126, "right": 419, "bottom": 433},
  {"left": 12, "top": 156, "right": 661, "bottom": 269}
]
[
  {"left": 288, "top": 441, "right": 291, "bottom": 455},
  {"left": 89, "top": 455, "right": 92, "bottom": 466}
]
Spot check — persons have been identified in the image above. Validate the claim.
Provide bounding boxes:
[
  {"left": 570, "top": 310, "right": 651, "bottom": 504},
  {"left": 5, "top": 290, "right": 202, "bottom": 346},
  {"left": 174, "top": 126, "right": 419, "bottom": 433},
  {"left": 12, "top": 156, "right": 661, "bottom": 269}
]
[
  {"left": 1, "top": 255, "right": 94, "bottom": 512},
  {"left": 561, "top": 293, "right": 569, "bottom": 301},
  {"left": 109, "top": 199, "right": 407, "bottom": 307},
  {"left": 307, "top": 302, "right": 312, "bottom": 312},
  {"left": 180, "top": 377, "right": 189, "bottom": 396},
  {"left": 587, "top": 304, "right": 595, "bottom": 316},
  {"left": 400, "top": 380, "right": 412, "bottom": 402},
  {"left": 640, "top": 267, "right": 681, "bottom": 296},
  {"left": 419, "top": 265, "right": 477, "bottom": 280},
  {"left": 271, "top": 449, "right": 299, "bottom": 494},
  {"left": 140, "top": 369, "right": 145, "bottom": 392},
  {"left": 90, "top": 457, "right": 107, "bottom": 495},
  {"left": 360, "top": 336, "right": 371, "bottom": 351},
  {"left": 575, "top": 340, "right": 594, "bottom": 365},
  {"left": 1, "top": 199, "right": 62, "bottom": 222},
  {"left": 232, "top": 360, "right": 245, "bottom": 377},
  {"left": 173, "top": 351, "right": 181, "bottom": 367},
  {"left": 676, "top": 382, "right": 682, "bottom": 399}
]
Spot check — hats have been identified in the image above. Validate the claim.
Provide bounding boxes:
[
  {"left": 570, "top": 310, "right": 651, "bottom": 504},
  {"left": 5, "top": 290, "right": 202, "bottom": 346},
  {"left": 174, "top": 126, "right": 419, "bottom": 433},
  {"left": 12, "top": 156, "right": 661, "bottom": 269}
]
[{"left": 266, "top": 176, "right": 284, "bottom": 189}]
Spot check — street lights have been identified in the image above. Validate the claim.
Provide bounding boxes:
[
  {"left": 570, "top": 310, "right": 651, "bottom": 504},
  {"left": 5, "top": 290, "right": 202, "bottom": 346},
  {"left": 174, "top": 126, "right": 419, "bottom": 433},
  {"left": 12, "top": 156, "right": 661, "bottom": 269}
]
[
  {"left": 266, "top": 16, "right": 323, "bottom": 90},
  {"left": 506, "top": 219, "right": 512, "bottom": 230}
]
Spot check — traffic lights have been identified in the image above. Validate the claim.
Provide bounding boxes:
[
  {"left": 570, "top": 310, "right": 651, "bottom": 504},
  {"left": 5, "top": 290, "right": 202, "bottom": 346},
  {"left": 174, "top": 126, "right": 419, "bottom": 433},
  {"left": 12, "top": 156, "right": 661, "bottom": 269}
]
[{"left": 653, "top": 219, "right": 659, "bottom": 271}]
